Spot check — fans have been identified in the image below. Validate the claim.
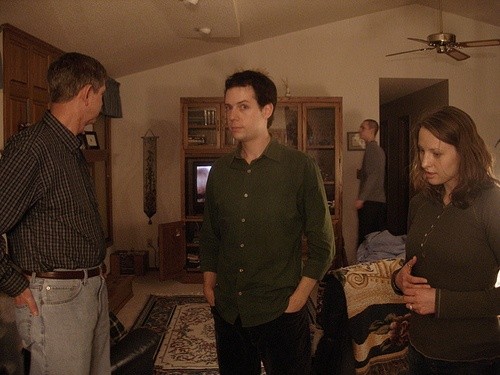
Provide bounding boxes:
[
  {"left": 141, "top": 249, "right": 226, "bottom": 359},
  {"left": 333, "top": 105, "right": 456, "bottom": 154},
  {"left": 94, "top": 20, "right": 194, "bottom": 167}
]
[{"left": 385, "top": 0, "right": 500, "bottom": 62}]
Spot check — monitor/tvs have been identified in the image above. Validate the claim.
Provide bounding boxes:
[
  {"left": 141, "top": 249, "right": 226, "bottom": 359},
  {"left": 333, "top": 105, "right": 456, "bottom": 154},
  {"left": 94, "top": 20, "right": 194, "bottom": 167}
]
[{"left": 185, "top": 156, "right": 220, "bottom": 216}]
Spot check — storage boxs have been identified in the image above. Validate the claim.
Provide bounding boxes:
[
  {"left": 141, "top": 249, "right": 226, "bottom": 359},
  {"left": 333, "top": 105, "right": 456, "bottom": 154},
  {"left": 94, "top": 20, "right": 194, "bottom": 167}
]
[{"left": 110, "top": 250, "right": 150, "bottom": 275}]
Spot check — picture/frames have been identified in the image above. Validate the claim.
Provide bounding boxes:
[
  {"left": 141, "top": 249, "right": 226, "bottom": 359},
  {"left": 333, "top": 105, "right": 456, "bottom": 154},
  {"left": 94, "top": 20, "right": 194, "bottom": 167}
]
[
  {"left": 84, "top": 131, "right": 100, "bottom": 150},
  {"left": 346, "top": 132, "right": 366, "bottom": 151}
]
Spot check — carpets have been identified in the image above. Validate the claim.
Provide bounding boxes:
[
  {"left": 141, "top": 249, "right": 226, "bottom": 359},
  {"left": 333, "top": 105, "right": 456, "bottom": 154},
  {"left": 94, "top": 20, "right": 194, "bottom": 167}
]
[{"left": 129, "top": 294, "right": 316, "bottom": 375}]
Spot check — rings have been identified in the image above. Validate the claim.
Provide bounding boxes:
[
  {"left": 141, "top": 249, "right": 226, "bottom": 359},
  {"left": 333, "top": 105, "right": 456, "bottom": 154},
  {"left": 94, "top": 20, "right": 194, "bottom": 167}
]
[
  {"left": 408, "top": 304, "right": 413, "bottom": 312},
  {"left": 406, "top": 264, "right": 412, "bottom": 271}
]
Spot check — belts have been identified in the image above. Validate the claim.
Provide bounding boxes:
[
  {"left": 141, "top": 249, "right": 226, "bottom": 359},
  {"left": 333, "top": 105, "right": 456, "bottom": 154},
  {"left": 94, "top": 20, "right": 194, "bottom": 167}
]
[{"left": 22, "top": 264, "right": 106, "bottom": 279}]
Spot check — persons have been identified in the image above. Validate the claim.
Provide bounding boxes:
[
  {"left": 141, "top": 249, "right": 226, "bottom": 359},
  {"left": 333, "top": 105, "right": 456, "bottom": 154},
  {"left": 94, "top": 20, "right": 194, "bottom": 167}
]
[
  {"left": 199, "top": 71, "right": 337, "bottom": 375},
  {"left": 0, "top": 51, "right": 111, "bottom": 375},
  {"left": 353, "top": 119, "right": 386, "bottom": 251},
  {"left": 391, "top": 105, "right": 500, "bottom": 375}
]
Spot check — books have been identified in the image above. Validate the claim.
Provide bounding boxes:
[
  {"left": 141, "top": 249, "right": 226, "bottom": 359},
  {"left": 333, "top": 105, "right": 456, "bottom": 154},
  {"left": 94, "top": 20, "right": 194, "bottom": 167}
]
[
  {"left": 189, "top": 107, "right": 215, "bottom": 125},
  {"left": 187, "top": 133, "right": 206, "bottom": 146}
]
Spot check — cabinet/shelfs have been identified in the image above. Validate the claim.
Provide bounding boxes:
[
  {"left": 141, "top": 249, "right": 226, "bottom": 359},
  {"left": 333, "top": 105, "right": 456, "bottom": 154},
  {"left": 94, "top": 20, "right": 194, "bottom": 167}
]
[
  {"left": 0, "top": 23, "right": 115, "bottom": 242},
  {"left": 179, "top": 96, "right": 343, "bottom": 284}
]
